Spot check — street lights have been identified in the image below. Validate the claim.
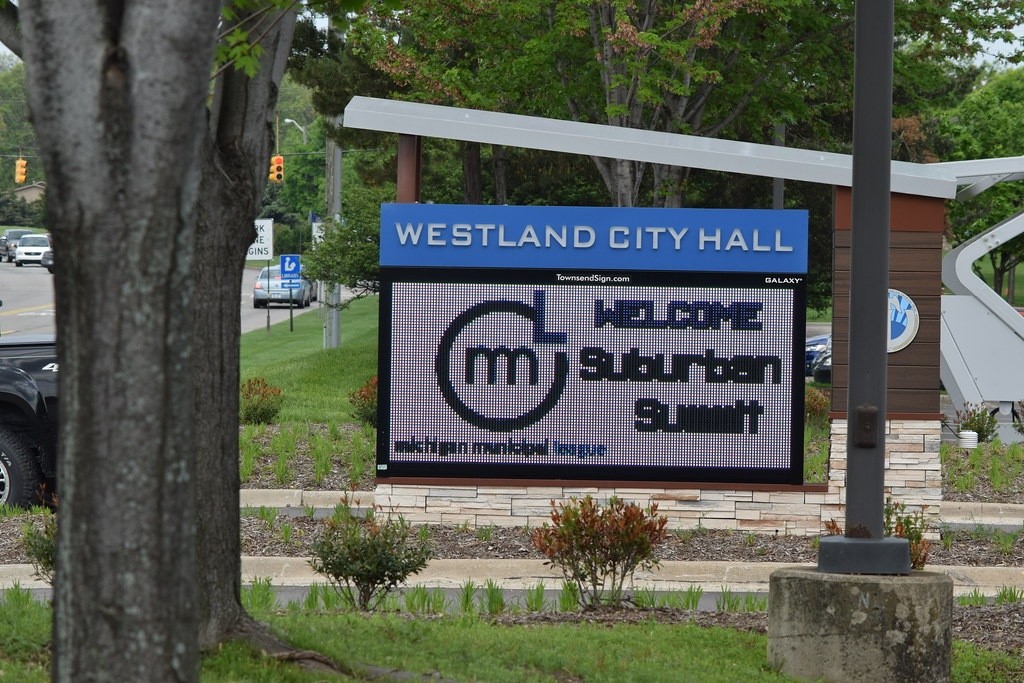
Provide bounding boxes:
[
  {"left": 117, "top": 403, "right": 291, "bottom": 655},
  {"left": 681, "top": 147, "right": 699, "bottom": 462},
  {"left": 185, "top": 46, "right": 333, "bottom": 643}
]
[{"left": 285, "top": 118, "right": 307, "bottom": 145}]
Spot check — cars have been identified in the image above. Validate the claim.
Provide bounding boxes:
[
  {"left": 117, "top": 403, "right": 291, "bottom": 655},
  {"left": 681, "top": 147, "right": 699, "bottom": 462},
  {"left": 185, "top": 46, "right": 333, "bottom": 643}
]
[
  {"left": 0, "top": 226, "right": 56, "bottom": 274},
  {"left": 806, "top": 331, "right": 832, "bottom": 383},
  {"left": 252, "top": 265, "right": 319, "bottom": 308},
  {"left": 0, "top": 336, "right": 58, "bottom": 506}
]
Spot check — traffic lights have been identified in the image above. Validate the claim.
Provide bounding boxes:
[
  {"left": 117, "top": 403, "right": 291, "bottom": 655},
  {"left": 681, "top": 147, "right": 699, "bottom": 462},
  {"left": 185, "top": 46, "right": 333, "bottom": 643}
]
[
  {"left": 269, "top": 155, "right": 284, "bottom": 183},
  {"left": 15, "top": 158, "right": 28, "bottom": 184}
]
